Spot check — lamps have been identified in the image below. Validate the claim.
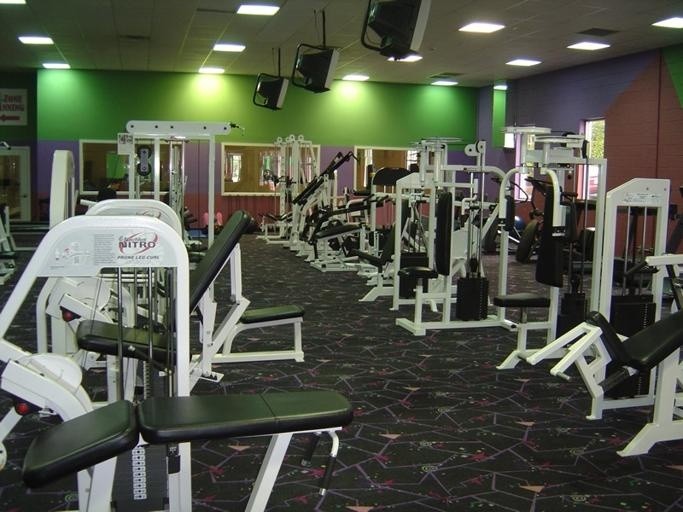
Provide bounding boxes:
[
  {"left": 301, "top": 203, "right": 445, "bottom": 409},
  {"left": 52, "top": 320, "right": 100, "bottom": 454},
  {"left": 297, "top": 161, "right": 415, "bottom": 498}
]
[
  {"left": 291, "top": 10, "right": 339, "bottom": 92},
  {"left": 253, "top": 49, "right": 289, "bottom": 110},
  {"left": 360, "top": 0, "right": 432, "bottom": 60}
]
